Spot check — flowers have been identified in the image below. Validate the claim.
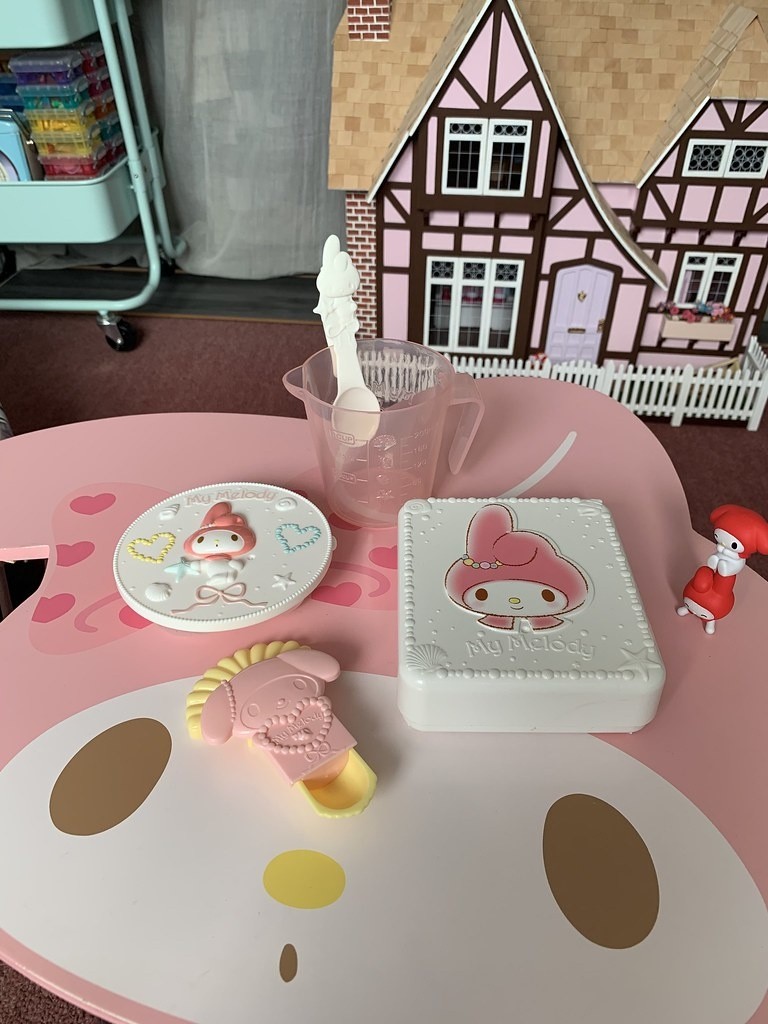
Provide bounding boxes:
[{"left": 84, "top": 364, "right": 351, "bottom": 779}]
[{"left": 657, "top": 300, "right": 734, "bottom": 325}]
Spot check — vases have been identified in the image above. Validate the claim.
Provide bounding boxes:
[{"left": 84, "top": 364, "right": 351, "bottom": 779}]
[{"left": 659, "top": 314, "right": 737, "bottom": 341}]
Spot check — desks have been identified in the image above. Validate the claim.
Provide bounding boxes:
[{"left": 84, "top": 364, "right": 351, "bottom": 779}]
[{"left": 0, "top": 373, "right": 768, "bottom": 1024}]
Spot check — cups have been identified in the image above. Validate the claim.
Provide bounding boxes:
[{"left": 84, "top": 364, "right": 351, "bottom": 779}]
[{"left": 282, "top": 337, "right": 486, "bottom": 531}]
